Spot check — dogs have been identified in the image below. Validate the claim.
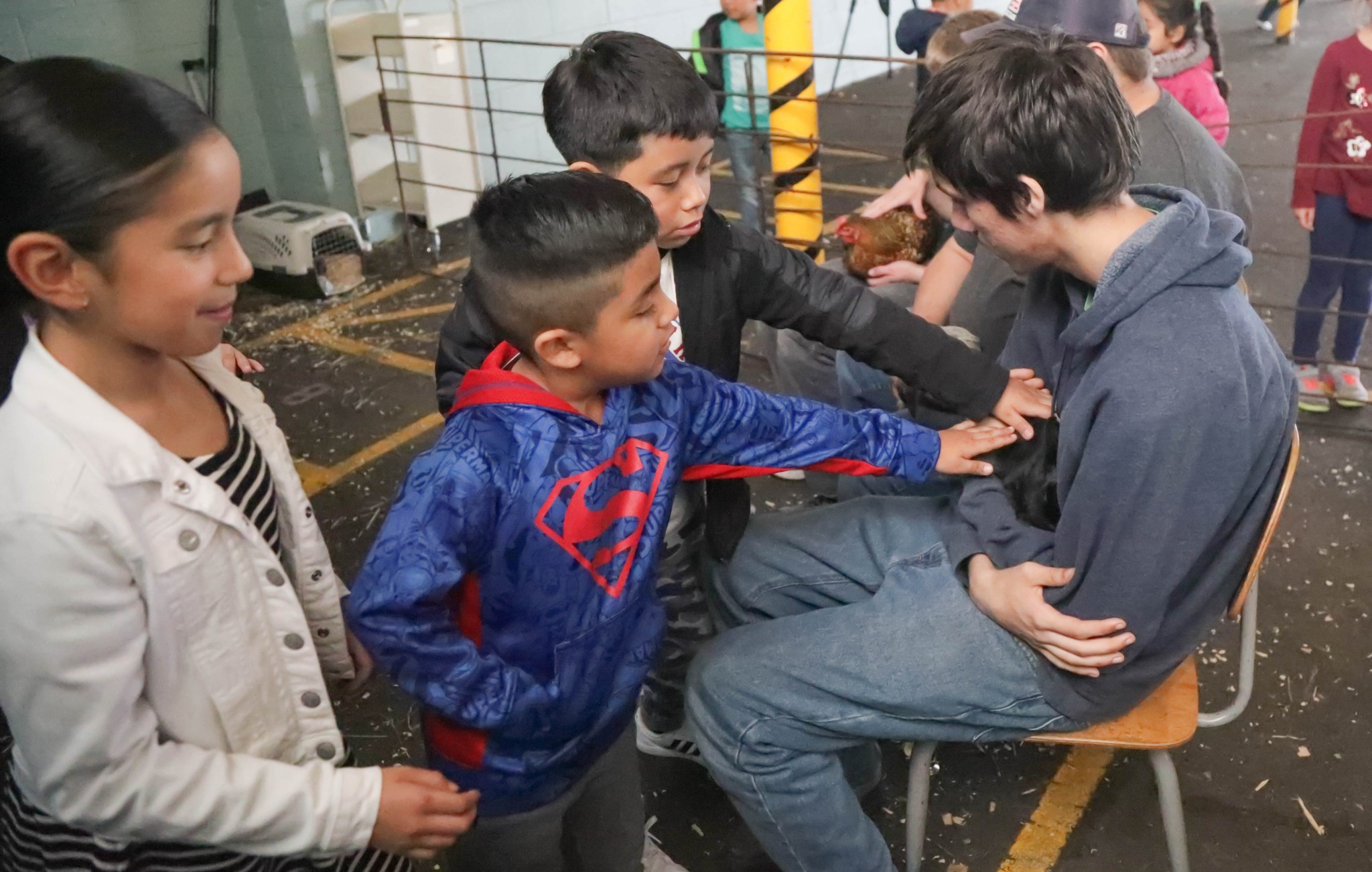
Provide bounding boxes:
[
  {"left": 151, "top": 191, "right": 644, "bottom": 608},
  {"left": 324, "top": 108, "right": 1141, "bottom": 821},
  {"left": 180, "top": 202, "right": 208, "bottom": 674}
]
[{"left": 895, "top": 323, "right": 1060, "bottom": 533}]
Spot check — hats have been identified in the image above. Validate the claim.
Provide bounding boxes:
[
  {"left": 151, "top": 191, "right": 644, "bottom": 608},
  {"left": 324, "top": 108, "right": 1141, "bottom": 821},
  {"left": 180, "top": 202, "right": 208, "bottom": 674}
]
[{"left": 961, "top": 0, "right": 1151, "bottom": 47}]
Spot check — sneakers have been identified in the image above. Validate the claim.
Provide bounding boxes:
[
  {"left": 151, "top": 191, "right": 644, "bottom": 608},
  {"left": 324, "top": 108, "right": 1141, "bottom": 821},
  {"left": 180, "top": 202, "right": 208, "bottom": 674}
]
[
  {"left": 636, "top": 706, "right": 709, "bottom": 768},
  {"left": 1328, "top": 363, "right": 1369, "bottom": 408},
  {"left": 1290, "top": 361, "right": 1330, "bottom": 412}
]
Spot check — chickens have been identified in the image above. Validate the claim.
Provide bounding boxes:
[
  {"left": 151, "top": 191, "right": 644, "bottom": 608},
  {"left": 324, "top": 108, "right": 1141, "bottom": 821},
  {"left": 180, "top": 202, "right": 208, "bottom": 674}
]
[{"left": 835, "top": 206, "right": 944, "bottom": 283}]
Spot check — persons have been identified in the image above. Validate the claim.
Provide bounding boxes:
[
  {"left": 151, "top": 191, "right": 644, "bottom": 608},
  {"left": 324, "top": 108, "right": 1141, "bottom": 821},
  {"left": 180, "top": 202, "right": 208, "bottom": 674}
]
[
  {"left": 1290, "top": 0, "right": 1372, "bottom": 415},
  {"left": 688, "top": 0, "right": 1298, "bottom": 872},
  {"left": 344, "top": 31, "right": 1053, "bottom": 872},
  {"left": 0, "top": 57, "right": 481, "bottom": 872}
]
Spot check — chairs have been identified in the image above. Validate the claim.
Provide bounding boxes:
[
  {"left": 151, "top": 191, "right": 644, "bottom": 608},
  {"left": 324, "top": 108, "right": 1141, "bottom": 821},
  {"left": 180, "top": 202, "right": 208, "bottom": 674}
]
[{"left": 905, "top": 424, "right": 1300, "bottom": 872}]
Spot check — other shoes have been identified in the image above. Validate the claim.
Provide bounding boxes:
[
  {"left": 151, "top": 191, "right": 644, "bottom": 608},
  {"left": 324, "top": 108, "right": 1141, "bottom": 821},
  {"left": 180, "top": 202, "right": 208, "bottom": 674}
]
[
  {"left": 1257, "top": 19, "right": 1272, "bottom": 30},
  {"left": 842, "top": 743, "right": 882, "bottom": 793}
]
